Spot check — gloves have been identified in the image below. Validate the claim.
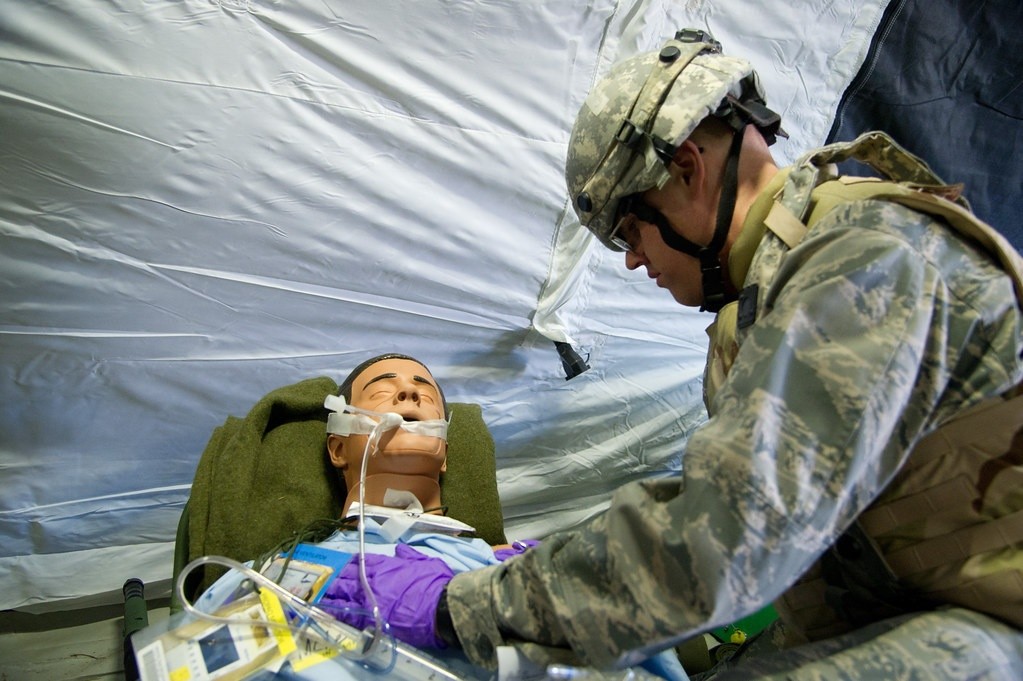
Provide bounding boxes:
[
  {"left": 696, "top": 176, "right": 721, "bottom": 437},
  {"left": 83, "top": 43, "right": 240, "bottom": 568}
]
[
  {"left": 494, "top": 536, "right": 541, "bottom": 562},
  {"left": 315, "top": 541, "right": 455, "bottom": 654}
]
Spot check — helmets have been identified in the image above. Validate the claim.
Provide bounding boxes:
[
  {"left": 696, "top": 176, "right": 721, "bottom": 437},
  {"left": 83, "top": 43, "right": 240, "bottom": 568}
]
[{"left": 565, "top": 26, "right": 767, "bottom": 253}]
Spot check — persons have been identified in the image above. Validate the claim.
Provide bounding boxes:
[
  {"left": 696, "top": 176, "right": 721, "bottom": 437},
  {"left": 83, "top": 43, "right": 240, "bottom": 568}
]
[
  {"left": 190, "top": 354, "right": 675, "bottom": 681},
  {"left": 282, "top": 28, "right": 1023, "bottom": 681}
]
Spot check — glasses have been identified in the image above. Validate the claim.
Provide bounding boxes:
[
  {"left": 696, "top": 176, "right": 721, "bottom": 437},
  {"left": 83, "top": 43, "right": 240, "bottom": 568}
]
[{"left": 608, "top": 146, "right": 703, "bottom": 256}]
